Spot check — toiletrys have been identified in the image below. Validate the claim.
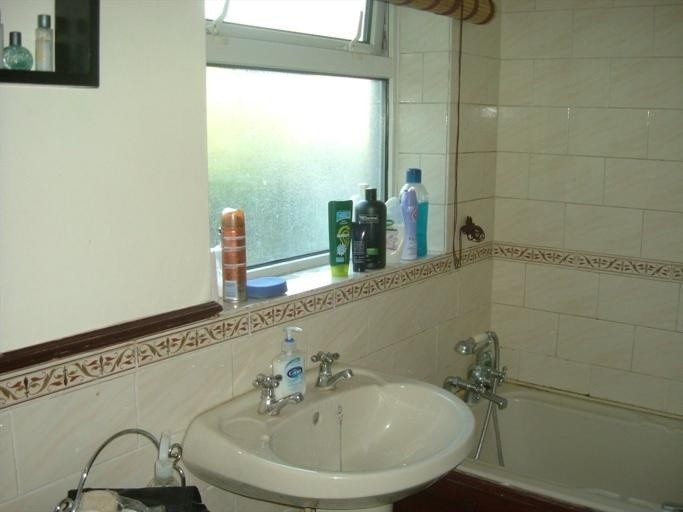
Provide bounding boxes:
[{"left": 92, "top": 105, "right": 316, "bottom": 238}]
[
  {"left": 34, "top": 13, "right": 54, "bottom": 71},
  {"left": 2, "top": 31, "right": 33, "bottom": 72}
]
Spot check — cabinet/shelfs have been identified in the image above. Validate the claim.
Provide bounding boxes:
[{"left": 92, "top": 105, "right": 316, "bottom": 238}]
[{"left": 0, "top": 1, "right": 98, "bottom": 88}]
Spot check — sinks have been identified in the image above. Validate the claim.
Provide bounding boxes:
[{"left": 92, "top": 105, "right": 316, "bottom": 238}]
[{"left": 178, "top": 362, "right": 476, "bottom": 508}]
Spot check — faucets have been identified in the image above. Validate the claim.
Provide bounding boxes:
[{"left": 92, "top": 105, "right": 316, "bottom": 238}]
[
  {"left": 252, "top": 373, "right": 304, "bottom": 415},
  {"left": 312, "top": 350, "right": 355, "bottom": 387},
  {"left": 482, "top": 390, "right": 507, "bottom": 411}
]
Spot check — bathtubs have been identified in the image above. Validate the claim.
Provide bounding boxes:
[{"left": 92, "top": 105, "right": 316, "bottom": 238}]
[{"left": 451, "top": 379, "right": 683, "bottom": 512}]
